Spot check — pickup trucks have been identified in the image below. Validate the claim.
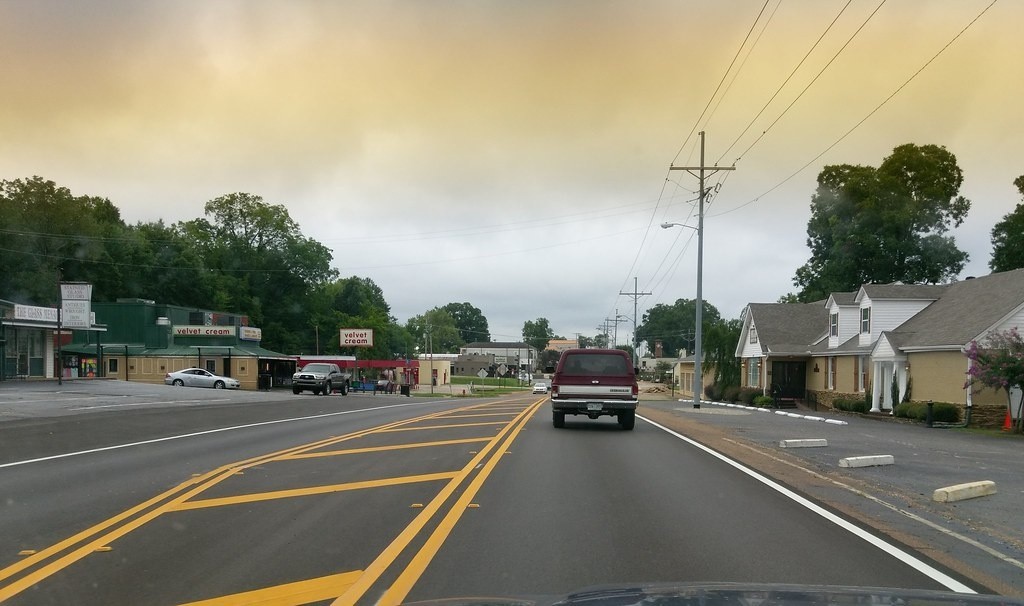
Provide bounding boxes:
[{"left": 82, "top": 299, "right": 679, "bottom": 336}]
[{"left": 292, "top": 363, "right": 352, "bottom": 396}]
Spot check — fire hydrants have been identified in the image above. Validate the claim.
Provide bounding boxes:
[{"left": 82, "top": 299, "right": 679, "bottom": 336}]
[{"left": 463, "top": 390, "right": 465, "bottom": 395}]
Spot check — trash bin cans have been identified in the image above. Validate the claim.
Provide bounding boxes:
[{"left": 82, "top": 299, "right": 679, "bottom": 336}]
[
  {"left": 401, "top": 383, "right": 410, "bottom": 396},
  {"left": 353, "top": 380, "right": 359, "bottom": 387}
]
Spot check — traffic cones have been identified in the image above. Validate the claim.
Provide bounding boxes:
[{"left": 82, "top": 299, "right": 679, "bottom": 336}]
[{"left": 1003, "top": 410, "right": 1012, "bottom": 430}]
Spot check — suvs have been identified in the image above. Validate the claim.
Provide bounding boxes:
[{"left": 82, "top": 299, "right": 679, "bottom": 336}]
[{"left": 546, "top": 348, "right": 640, "bottom": 430}]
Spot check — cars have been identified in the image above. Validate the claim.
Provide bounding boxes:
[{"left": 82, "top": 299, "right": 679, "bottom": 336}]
[
  {"left": 164, "top": 368, "right": 240, "bottom": 389},
  {"left": 533, "top": 382, "right": 548, "bottom": 395}
]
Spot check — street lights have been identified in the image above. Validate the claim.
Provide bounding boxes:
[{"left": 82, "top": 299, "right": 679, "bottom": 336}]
[
  {"left": 616, "top": 313, "right": 636, "bottom": 374},
  {"left": 661, "top": 221, "right": 705, "bottom": 408}
]
